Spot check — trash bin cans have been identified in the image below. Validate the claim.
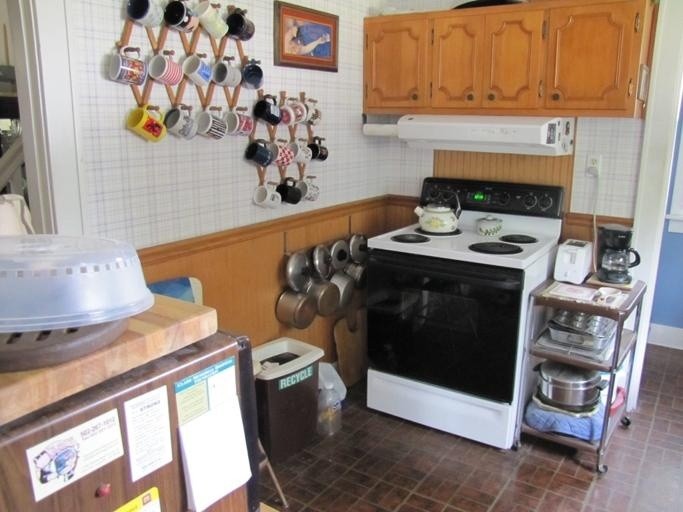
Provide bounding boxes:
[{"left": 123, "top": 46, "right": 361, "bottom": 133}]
[{"left": 251, "top": 336, "right": 324, "bottom": 464}]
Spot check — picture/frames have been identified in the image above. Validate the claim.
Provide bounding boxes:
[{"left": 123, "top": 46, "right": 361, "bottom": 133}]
[{"left": 271, "top": 0, "right": 340, "bottom": 72}]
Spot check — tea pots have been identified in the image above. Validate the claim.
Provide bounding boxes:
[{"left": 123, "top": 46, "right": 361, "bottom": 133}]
[{"left": 410, "top": 189, "right": 462, "bottom": 235}]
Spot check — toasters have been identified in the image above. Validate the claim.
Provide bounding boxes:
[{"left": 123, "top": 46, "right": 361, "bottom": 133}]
[{"left": 553, "top": 236, "right": 595, "bottom": 283}]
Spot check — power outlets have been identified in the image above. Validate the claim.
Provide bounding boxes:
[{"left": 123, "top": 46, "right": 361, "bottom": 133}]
[{"left": 583, "top": 151, "right": 603, "bottom": 180}]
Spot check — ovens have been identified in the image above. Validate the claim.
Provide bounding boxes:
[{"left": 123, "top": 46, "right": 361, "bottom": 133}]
[{"left": 365, "top": 261, "right": 523, "bottom": 451}]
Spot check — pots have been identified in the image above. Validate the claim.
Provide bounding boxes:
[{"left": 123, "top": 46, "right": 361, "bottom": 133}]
[
  {"left": 533, "top": 361, "right": 609, "bottom": 407},
  {"left": 274, "top": 263, "right": 365, "bottom": 331}
]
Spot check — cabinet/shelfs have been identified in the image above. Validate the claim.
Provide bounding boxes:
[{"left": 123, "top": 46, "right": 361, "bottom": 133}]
[
  {"left": 513, "top": 273, "right": 652, "bottom": 481},
  {"left": 539, "top": 1, "right": 660, "bottom": 122},
  {"left": 358, "top": 5, "right": 433, "bottom": 114},
  {"left": 427, "top": 2, "right": 546, "bottom": 117}
]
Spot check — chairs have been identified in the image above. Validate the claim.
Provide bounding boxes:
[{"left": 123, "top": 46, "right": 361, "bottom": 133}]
[{"left": 148, "top": 278, "right": 296, "bottom": 512}]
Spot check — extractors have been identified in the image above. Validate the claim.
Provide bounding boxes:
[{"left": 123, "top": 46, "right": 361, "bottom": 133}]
[{"left": 394, "top": 112, "right": 575, "bottom": 155}]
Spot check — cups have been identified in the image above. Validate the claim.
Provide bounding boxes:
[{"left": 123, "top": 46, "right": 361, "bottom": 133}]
[
  {"left": 279, "top": 96, "right": 308, "bottom": 126},
  {"left": 268, "top": 137, "right": 295, "bottom": 166},
  {"left": 599, "top": 286, "right": 623, "bottom": 304},
  {"left": 226, "top": 8, "right": 256, "bottom": 39},
  {"left": 162, "top": 103, "right": 197, "bottom": 140},
  {"left": 308, "top": 136, "right": 329, "bottom": 161},
  {"left": 211, "top": 58, "right": 242, "bottom": 88},
  {"left": 182, "top": 52, "right": 212, "bottom": 85},
  {"left": 276, "top": 177, "right": 302, "bottom": 205},
  {"left": 252, "top": 94, "right": 282, "bottom": 126},
  {"left": 297, "top": 174, "right": 320, "bottom": 202},
  {"left": 193, "top": 1, "right": 229, "bottom": 39},
  {"left": 288, "top": 137, "right": 313, "bottom": 164},
  {"left": 224, "top": 105, "right": 253, "bottom": 136},
  {"left": 109, "top": 46, "right": 147, "bottom": 86},
  {"left": 161, "top": 1, "right": 199, "bottom": 32},
  {"left": 148, "top": 50, "right": 183, "bottom": 87},
  {"left": 245, "top": 138, "right": 273, "bottom": 167},
  {"left": 253, "top": 179, "right": 281, "bottom": 208},
  {"left": 124, "top": 104, "right": 168, "bottom": 143},
  {"left": 195, "top": 105, "right": 228, "bottom": 141},
  {"left": 243, "top": 58, "right": 264, "bottom": 90},
  {"left": 304, "top": 98, "right": 322, "bottom": 125},
  {"left": 126, "top": 1, "right": 165, "bottom": 27}
]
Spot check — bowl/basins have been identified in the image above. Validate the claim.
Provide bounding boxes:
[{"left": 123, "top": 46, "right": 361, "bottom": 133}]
[{"left": 0, "top": 232, "right": 154, "bottom": 334}]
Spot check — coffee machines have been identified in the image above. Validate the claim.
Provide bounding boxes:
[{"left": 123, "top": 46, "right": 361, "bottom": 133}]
[{"left": 596, "top": 222, "right": 641, "bottom": 283}]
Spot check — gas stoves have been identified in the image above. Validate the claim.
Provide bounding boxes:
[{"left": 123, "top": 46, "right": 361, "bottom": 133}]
[{"left": 366, "top": 222, "right": 559, "bottom": 268}]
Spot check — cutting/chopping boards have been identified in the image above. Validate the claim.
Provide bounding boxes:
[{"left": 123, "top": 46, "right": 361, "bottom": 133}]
[{"left": 0, "top": 292, "right": 217, "bottom": 430}]
